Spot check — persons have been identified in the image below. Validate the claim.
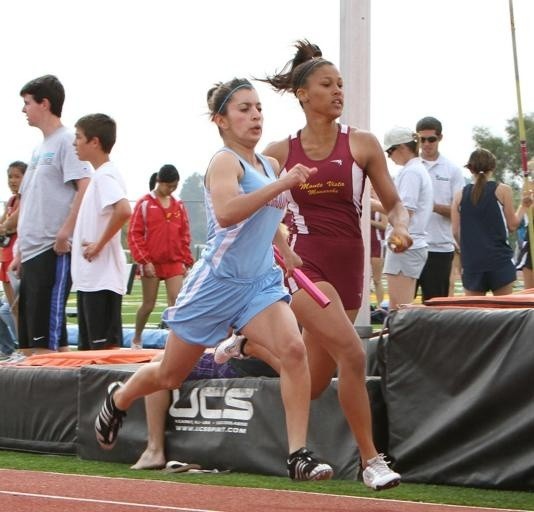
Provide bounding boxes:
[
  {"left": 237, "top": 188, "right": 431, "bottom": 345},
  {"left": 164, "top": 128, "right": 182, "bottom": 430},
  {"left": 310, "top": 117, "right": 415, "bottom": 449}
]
[
  {"left": 215, "top": 40, "right": 412, "bottom": 492},
  {"left": 128, "top": 350, "right": 279, "bottom": 470},
  {"left": 127, "top": 164, "right": 194, "bottom": 350},
  {"left": 94, "top": 79, "right": 333, "bottom": 481},
  {"left": 8, "top": 75, "right": 92, "bottom": 353},
  {"left": 71, "top": 113, "right": 132, "bottom": 348},
  {"left": 370, "top": 117, "right": 534, "bottom": 311},
  {"left": 0, "top": 161, "right": 32, "bottom": 363}
]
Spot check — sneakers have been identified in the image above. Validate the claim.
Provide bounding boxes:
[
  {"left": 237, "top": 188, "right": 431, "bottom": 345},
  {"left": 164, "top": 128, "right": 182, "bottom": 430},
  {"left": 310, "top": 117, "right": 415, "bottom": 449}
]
[
  {"left": 285, "top": 447, "right": 333, "bottom": 481},
  {"left": 130, "top": 338, "right": 143, "bottom": 348},
  {"left": 95, "top": 380, "right": 127, "bottom": 449},
  {"left": 213, "top": 334, "right": 246, "bottom": 364},
  {"left": 362, "top": 453, "right": 402, "bottom": 491}
]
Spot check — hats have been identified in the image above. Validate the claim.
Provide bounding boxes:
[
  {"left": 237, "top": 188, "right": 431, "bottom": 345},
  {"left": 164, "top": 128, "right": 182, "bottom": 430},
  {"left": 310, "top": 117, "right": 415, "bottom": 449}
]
[{"left": 382, "top": 126, "right": 415, "bottom": 152}]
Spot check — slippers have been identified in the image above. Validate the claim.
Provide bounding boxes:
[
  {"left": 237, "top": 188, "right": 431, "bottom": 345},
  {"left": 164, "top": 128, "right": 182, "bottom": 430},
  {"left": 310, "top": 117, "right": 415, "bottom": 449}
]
[{"left": 163, "top": 460, "right": 230, "bottom": 475}]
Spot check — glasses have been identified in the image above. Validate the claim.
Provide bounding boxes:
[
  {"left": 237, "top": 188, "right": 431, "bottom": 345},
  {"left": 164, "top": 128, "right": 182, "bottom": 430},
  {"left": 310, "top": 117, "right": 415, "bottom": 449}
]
[
  {"left": 387, "top": 147, "right": 395, "bottom": 156},
  {"left": 420, "top": 137, "right": 437, "bottom": 143}
]
